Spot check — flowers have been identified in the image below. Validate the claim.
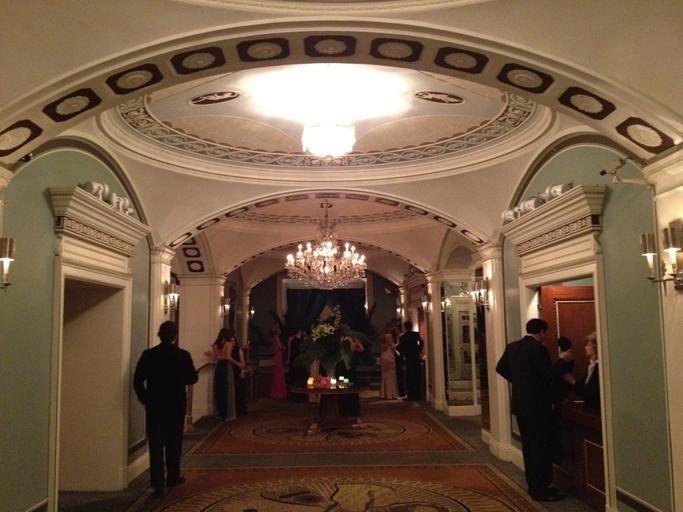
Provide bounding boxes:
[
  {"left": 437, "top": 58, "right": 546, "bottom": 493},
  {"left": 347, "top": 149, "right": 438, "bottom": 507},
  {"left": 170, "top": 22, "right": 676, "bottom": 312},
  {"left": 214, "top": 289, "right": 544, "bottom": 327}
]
[{"left": 302, "top": 304, "right": 371, "bottom": 367}]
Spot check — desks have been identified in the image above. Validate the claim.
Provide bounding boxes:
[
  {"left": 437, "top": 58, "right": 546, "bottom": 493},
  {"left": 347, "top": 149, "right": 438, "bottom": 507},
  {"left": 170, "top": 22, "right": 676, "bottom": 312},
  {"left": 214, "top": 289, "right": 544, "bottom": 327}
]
[{"left": 290, "top": 389, "right": 360, "bottom": 427}]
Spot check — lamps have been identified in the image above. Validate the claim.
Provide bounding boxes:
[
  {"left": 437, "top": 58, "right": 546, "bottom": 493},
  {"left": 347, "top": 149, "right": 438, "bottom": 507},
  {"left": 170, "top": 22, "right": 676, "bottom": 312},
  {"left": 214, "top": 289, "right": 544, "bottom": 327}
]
[
  {"left": 224, "top": 297, "right": 231, "bottom": 315},
  {"left": 0, "top": 237, "right": 15, "bottom": 290},
  {"left": 250, "top": 305, "right": 255, "bottom": 319},
  {"left": 639, "top": 228, "right": 683, "bottom": 296},
  {"left": 421, "top": 295, "right": 428, "bottom": 313},
  {"left": 163, "top": 283, "right": 180, "bottom": 314},
  {"left": 474, "top": 279, "right": 490, "bottom": 310},
  {"left": 285, "top": 202, "right": 367, "bottom": 290},
  {"left": 396, "top": 302, "right": 402, "bottom": 319}
]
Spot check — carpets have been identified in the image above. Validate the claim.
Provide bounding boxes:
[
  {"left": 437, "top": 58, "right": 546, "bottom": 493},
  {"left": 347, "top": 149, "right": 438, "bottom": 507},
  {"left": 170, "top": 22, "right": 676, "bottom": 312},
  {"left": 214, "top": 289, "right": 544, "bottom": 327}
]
[
  {"left": 183, "top": 400, "right": 479, "bottom": 455},
  {"left": 124, "top": 464, "right": 551, "bottom": 512}
]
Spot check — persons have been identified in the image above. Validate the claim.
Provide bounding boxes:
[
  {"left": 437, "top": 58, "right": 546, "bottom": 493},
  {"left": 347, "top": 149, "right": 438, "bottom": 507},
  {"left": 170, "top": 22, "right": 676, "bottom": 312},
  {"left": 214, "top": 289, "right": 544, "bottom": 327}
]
[
  {"left": 551, "top": 337, "right": 578, "bottom": 380},
  {"left": 212, "top": 328, "right": 305, "bottom": 421},
  {"left": 566, "top": 333, "right": 601, "bottom": 418},
  {"left": 375, "top": 319, "right": 424, "bottom": 400},
  {"left": 132, "top": 320, "right": 199, "bottom": 493},
  {"left": 495, "top": 318, "right": 574, "bottom": 503}
]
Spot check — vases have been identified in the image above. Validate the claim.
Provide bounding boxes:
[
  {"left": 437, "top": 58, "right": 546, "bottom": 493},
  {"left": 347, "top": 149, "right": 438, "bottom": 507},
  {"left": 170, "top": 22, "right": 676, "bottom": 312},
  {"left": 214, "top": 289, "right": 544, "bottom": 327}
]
[{"left": 325, "top": 368, "right": 336, "bottom": 379}]
